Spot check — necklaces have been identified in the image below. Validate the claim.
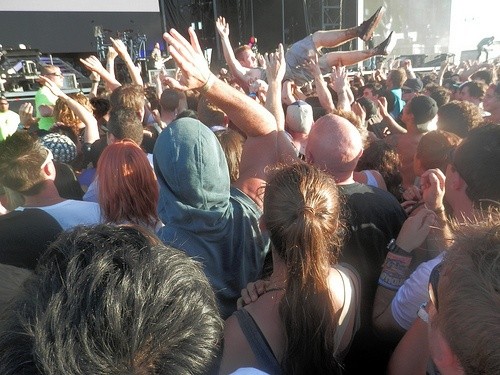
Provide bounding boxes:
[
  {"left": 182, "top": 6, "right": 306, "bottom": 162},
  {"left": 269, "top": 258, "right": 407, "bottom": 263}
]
[
  {"left": 259, "top": 287, "right": 287, "bottom": 299},
  {"left": 23, "top": 198, "right": 62, "bottom": 205}
]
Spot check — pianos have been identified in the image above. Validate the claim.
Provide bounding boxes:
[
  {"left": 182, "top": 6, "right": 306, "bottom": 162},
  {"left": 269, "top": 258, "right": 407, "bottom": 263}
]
[{"left": 0, "top": 47, "right": 42, "bottom": 91}]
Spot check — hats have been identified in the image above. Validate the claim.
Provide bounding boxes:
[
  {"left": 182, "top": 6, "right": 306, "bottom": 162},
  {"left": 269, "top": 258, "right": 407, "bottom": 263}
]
[
  {"left": 403, "top": 78, "right": 423, "bottom": 92},
  {"left": 410, "top": 94, "right": 438, "bottom": 118},
  {"left": 287, "top": 100, "right": 313, "bottom": 132},
  {"left": 41, "top": 132, "right": 77, "bottom": 161}
]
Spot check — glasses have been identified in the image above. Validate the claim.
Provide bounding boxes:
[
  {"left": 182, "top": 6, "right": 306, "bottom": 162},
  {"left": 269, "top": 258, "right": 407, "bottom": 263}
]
[
  {"left": 45, "top": 72, "right": 62, "bottom": 75},
  {"left": 401, "top": 88, "right": 416, "bottom": 92}
]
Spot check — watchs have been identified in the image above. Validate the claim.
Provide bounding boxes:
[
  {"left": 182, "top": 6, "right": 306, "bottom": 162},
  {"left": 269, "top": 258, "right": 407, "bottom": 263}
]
[
  {"left": 17, "top": 123, "right": 29, "bottom": 130},
  {"left": 387, "top": 239, "right": 412, "bottom": 257}
]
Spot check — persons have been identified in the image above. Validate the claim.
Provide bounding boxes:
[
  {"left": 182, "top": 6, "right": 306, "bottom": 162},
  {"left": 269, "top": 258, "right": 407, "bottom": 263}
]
[{"left": 0, "top": 5, "right": 500, "bottom": 374}]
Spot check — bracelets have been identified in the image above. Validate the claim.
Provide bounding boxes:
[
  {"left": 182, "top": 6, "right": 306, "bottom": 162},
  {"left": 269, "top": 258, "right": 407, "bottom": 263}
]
[
  {"left": 337, "top": 90, "right": 345, "bottom": 96},
  {"left": 196, "top": 73, "right": 215, "bottom": 94},
  {"left": 435, "top": 205, "right": 445, "bottom": 215}
]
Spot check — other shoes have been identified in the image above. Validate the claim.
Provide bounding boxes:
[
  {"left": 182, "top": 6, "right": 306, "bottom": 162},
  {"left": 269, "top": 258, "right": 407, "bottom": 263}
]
[
  {"left": 377, "top": 30, "right": 396, "bottom": 55},
  {"left": 357, "top": 6, "right": 386, "bottom": 41}
]
